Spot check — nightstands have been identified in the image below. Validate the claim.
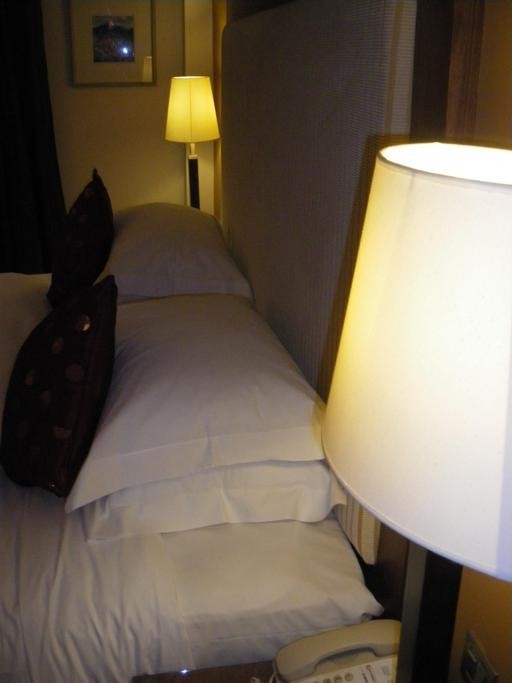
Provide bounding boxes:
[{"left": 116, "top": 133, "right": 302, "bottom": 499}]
[{"left": 131, "top": 660, "right": 283, "bottom": 683}]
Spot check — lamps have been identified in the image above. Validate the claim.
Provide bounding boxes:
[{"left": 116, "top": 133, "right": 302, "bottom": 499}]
[
  {"left": 165, "top": 76, "right": 220, "bottom": 209},
  {"left": 323, "top": 144, "right": 511, "bottom": 683}
]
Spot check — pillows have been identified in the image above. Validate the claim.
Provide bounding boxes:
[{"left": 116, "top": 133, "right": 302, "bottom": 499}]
[
  {"left": 79, "top": 462, "right": 341, "bottom": 544},
  {"left": 64, "top": 300, "right": 329, "bottom": 514},
  {"left": 93, "top": 201, "right": 253, "bottom": 305},
  {"left": 46, "top": 168, "right": 115, "bottom": 304},
  {"left": 3, "top": 276, "right": 120, "bottom": 493}
]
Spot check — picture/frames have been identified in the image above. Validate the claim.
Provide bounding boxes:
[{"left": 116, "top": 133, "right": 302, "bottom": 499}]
[{"left": 64, "top": 0, "right": 157, "bottom": 88}]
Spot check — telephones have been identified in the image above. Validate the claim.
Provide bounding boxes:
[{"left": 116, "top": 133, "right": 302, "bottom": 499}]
[{"left": 252, "top": 619, "right": 402, "bottom": 683}]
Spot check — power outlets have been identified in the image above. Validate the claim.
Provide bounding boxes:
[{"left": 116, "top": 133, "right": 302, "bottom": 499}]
[{"left": 459, "top": 632, "right": 497, "bottom": 683}]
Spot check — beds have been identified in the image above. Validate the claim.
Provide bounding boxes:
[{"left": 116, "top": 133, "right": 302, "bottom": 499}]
[{"left": 0, "top": 0, "right": 448, "bottom": 683}]
